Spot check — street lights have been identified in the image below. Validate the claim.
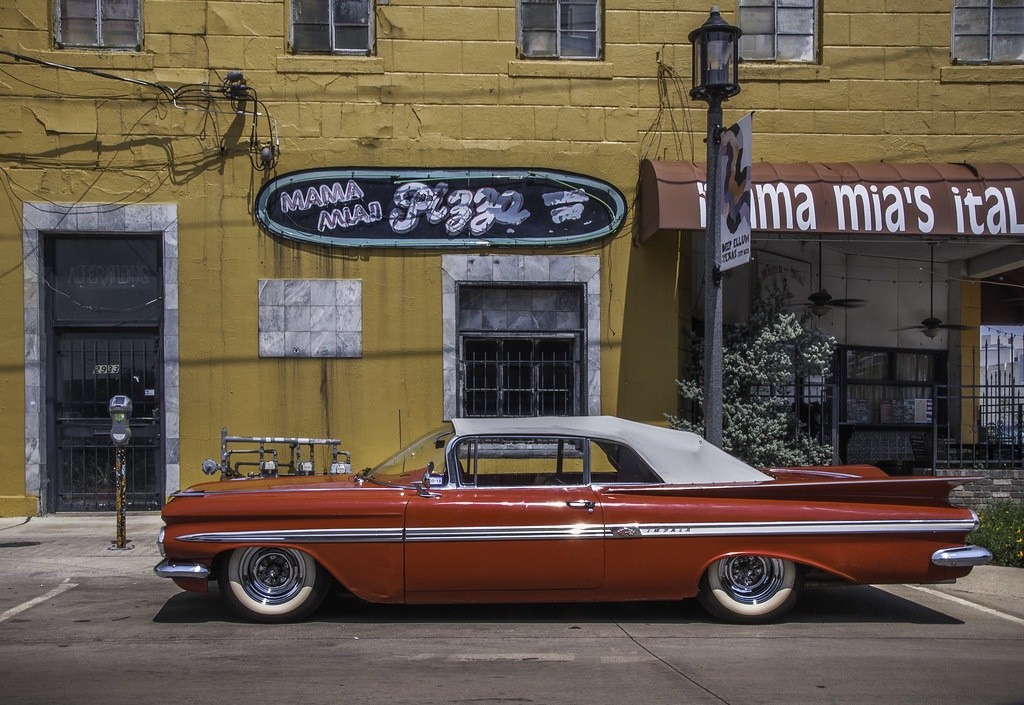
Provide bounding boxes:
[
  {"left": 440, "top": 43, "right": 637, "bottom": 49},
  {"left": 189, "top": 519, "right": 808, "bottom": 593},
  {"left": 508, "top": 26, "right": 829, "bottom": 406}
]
[{"left": 686, "top": 3, "right": 745, "bottom": 448}]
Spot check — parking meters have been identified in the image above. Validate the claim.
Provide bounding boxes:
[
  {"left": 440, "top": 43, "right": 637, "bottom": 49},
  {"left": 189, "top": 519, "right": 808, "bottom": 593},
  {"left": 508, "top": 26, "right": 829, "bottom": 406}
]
[{"left": 108, "top": 395, "right": 132, "bottom": 549}]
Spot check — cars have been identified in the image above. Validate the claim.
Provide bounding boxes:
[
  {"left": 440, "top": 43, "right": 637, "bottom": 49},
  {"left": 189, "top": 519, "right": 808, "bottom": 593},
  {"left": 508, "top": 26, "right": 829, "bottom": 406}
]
[{"left": 151, "top": 413, "right": 992, "bottom": 627}]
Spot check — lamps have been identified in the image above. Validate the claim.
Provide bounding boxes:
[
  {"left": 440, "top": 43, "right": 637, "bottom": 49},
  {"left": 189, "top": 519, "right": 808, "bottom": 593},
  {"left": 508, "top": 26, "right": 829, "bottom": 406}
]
[
  {"left": 810, "top": 304, "right": 831, "bottom": 317},
  {"left": 921, "top": 327, "right": 940, "bottom": 338}
]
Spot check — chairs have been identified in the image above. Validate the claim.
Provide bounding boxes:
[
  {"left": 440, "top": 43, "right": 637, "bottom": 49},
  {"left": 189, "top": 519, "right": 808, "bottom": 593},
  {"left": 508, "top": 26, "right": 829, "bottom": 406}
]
[
  {"left": 925, "top": 432, "right": 955, "bottom": 469},
  {"left": 910, "top": 433, "right": 932, "bottom": 470}
]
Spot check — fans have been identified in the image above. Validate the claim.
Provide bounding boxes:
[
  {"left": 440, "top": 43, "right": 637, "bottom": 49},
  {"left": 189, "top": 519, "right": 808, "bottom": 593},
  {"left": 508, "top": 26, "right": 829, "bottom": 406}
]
[
  {"left": 888, "top": 240, "right": 978, "bottom": 330},
  {"left": 787, "top": 229, "right": 867, "bottom": 308}
]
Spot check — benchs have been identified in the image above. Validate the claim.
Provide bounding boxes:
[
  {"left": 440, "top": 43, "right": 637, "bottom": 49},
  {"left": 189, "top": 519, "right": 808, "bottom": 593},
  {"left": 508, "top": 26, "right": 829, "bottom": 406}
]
[{"left": 943, "top": 439, "right": 972, "bottom": 460}]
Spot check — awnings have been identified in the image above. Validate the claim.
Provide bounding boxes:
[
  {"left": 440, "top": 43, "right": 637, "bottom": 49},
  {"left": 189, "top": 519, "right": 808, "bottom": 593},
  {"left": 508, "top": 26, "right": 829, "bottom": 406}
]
[{"left": 639, "top": 157, "right": 1024, "bottom": 245}]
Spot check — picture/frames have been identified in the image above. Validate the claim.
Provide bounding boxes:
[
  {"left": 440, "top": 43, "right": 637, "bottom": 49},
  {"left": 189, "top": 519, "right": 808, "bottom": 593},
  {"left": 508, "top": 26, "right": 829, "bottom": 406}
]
[{"left": 756, "top": 249, "right": 812, "bottom": 305}]
